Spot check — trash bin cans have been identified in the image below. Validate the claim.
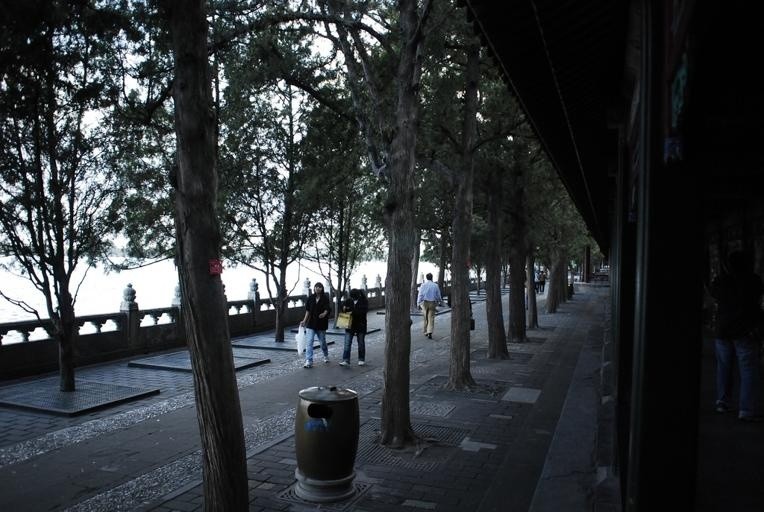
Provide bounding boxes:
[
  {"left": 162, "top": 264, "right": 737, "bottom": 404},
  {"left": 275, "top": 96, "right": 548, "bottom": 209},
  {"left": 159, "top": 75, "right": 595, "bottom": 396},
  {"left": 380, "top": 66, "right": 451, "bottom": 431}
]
[{"left": 294, "top": 385, "right": 359, "bottom": 503}]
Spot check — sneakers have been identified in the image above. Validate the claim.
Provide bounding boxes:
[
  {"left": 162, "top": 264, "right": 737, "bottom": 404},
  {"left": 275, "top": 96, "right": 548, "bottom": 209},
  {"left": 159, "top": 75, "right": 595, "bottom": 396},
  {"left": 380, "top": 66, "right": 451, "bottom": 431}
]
[
  {"left": 303, "top": 361, "right": 313, "bottom": 368},
  {"left": 339, "top": 360, "right": 351, "bottom": 367},
  {"left": 357, "top": 360, "right": 366, "bottom": 366},
  {"left": 323, "top": 357, "right": 331, "bottom": 363}
]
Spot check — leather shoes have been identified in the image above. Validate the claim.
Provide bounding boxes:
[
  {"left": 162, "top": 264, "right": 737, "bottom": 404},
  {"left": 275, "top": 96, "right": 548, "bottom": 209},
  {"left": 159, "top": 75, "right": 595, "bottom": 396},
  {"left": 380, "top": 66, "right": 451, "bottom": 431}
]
[{"left": 424, "top": 333, "right": 433, "bottom": 339}]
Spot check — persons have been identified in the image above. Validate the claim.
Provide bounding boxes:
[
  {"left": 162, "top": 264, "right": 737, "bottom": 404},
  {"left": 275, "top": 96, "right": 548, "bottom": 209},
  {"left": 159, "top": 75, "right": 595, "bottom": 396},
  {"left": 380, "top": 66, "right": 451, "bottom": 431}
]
[
  {"left": 300, "top": 282, "right": 331, "bottom": 368},
  {"left": 417, "top": 273, "right": 442, "bottom": 339},
  {"left": 339, "top": 288, "right": 369, "bottom": 366},
  {"left": 534, "top": 270, "right": 549, "bottom": 293}
]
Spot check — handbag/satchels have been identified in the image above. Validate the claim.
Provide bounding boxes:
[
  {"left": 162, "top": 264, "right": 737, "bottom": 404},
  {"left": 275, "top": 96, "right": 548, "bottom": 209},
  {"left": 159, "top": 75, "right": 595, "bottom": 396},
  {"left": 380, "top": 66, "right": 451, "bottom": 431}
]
[
  {"left": 471, "top": 315, "right": 476, "bottom": 329},
  {"left": 336, "top": 312, "right": 354, "bottom": 329}
]
